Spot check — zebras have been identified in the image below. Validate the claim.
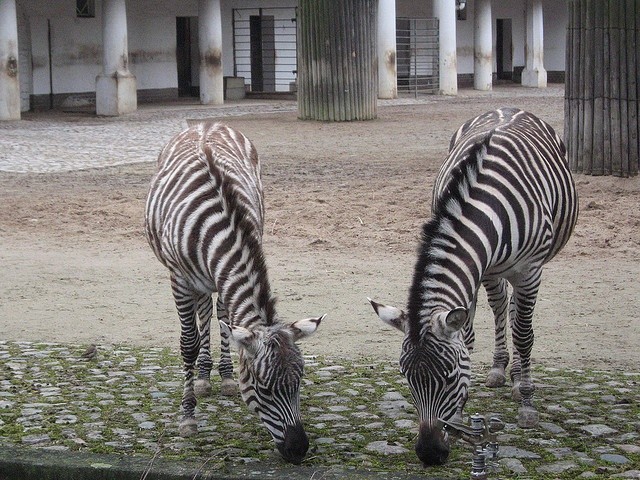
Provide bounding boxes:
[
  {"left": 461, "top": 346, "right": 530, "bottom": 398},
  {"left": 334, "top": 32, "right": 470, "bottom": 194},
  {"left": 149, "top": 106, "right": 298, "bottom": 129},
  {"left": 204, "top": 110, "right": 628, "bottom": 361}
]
[
  {"left": 365, "top": 107, "right": 580, "bottom": 469},
  {"left": 143, "top": 119, "right": 329, "bottom": 466}
]
